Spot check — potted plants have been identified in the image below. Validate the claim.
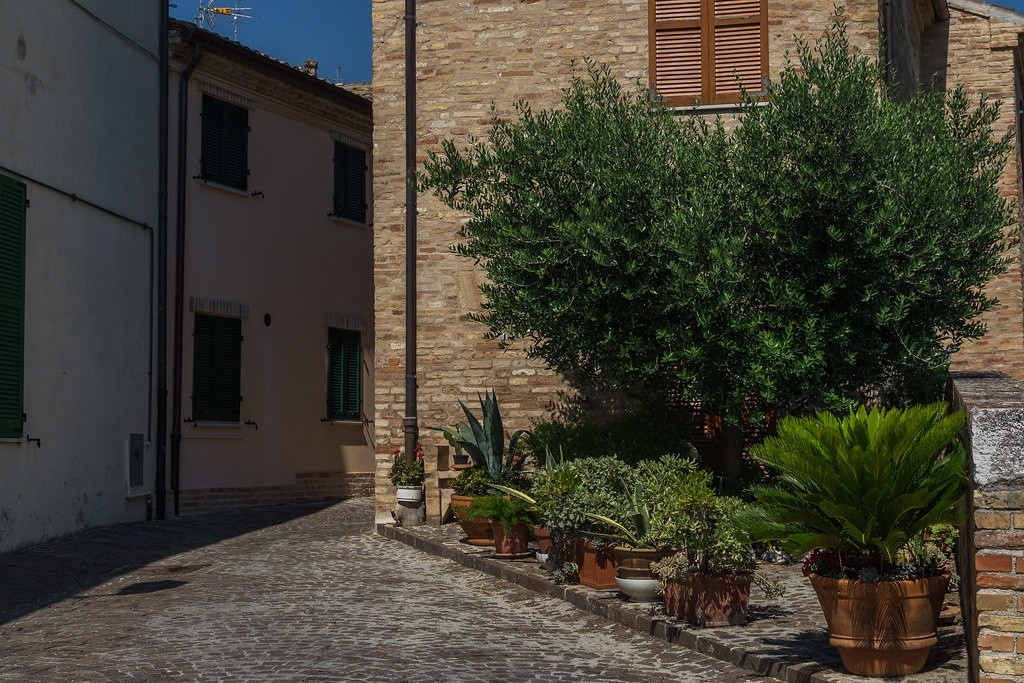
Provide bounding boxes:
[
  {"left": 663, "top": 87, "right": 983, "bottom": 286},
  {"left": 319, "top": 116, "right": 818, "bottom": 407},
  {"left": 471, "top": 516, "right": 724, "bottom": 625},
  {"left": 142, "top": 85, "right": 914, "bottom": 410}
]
[
  {"left": 644, "top": 455, "right": 786, "bottom": 626},
  {"left": 573, "top": 477, "right": 684, "bottom": 604},
  {"left": 534, "top": 455, "right": 647, "bottom": 590},
  {"left": 443, "top": 423, "right": 473, "bottom": 471},
  {"left": 532, "top": 447, "right": 580, "bottom": 569},
  {"left": 459, "top": 492, "right": 536, "bottom": 559},
  {"left": 451, "top": 467, "right": 512, "bottom": 546},
  {"left": 502, "top": 435, "right": 526, "bottom": 465},
  {"left": 743, "top": 400, "right": 975, "bottom": 678}
]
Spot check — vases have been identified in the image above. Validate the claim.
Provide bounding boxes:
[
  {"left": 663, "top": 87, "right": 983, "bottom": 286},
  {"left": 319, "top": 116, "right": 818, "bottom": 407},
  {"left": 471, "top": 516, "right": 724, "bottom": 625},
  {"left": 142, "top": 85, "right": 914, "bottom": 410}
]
[{"left": 396, "top": 485, "right": 422, "bottom": 504}]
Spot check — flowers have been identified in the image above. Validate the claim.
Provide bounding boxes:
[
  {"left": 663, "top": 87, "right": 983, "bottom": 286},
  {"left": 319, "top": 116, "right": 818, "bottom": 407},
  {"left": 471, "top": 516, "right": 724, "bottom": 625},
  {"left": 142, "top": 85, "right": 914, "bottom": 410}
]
[{"left": 388, "top": 441, "right": 430, "bottom": 487}]
